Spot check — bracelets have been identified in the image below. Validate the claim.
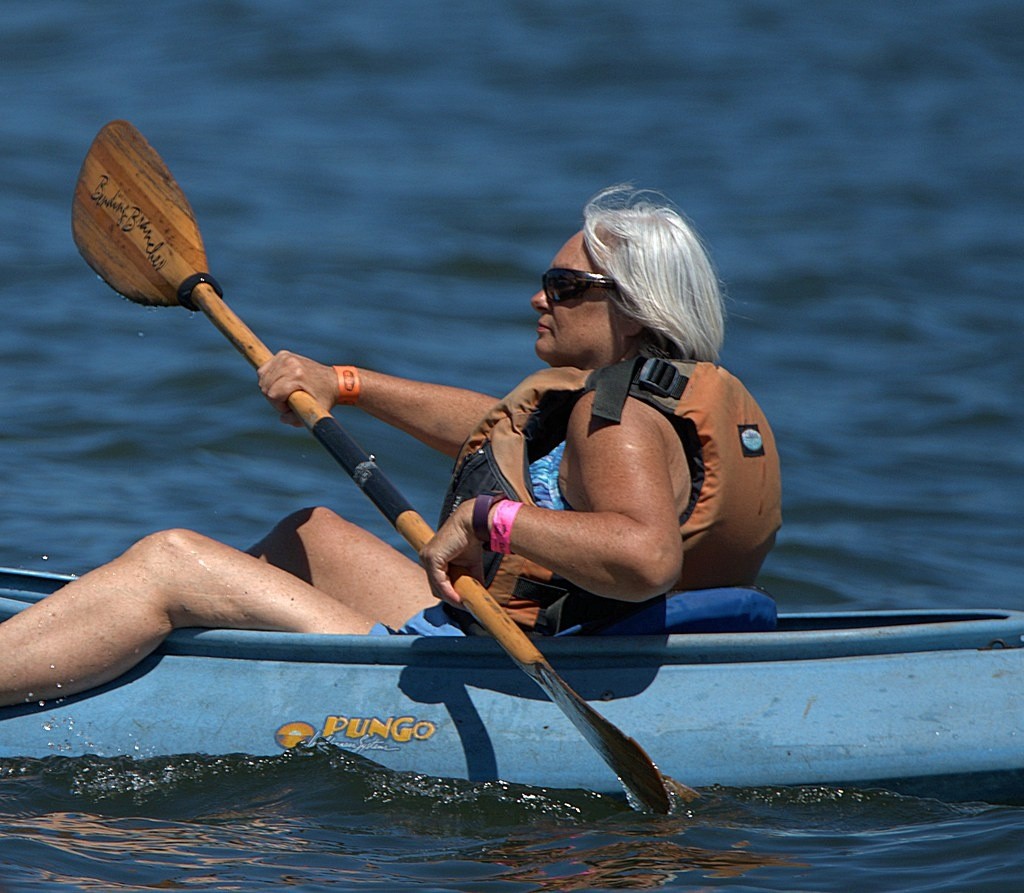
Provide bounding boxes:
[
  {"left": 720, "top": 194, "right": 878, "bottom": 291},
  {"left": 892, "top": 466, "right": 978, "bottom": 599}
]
[
  {"left": 334, "top": 364, "right": 361, "bottom": 406},
  {"left": 472, "top": 492, "right": 524, "bottom": 556}
]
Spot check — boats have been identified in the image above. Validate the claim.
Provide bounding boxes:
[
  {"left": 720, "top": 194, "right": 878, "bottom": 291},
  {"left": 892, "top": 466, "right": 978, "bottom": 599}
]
[{"left": 0, "top": 568, "right": 1024, "bottom": 795}]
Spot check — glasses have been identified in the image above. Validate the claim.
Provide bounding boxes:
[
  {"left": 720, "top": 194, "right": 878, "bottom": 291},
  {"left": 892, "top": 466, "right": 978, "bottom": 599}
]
[{"left": 541, "top": 268, "right": 619, "bottom": 301}]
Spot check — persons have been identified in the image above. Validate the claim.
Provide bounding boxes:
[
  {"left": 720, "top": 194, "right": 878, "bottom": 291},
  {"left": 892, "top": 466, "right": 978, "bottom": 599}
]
[{"left": 0, "top": 182, "right": 724, "bottom": 706}]
